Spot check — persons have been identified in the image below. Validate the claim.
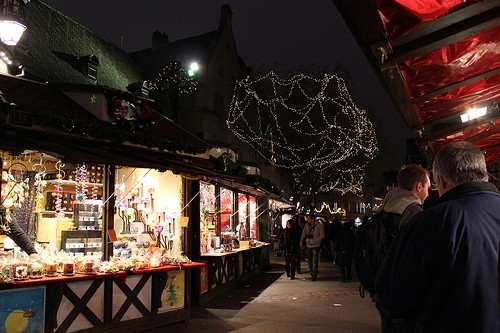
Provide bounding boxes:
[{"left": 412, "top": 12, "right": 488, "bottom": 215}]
[
  {"left": 373, "top": 141, "right": 500, "bottom": 333},
  {"left": 300, "top": 214, "right": 324, "bottom": 281},
  {"left": 273, "top": 213, "right": 371, "bottom": 281},
  {"left": 278, "top": 219, "right": 304, "bottom": 280},
  {"left": 337, "top": 222, "right": 356, "bottom": 282},
  {"left": 354, "top": 164, "right": 432, "bottom": 333},
  {"left": 0, "top": 187, "right": 42, "bottom": 263}
]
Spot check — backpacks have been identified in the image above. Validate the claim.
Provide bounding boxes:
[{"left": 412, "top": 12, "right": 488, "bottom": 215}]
[{"left": 354, "top": 201, "right": 421, "bottom": 299}]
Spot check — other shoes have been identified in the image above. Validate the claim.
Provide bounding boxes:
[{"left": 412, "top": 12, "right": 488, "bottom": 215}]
[
  {"left": 311, "top": 276, "right": 316, "bottom": 281},
  {"left": 291, "top": 275, "right": 295, "bottom": 279}
]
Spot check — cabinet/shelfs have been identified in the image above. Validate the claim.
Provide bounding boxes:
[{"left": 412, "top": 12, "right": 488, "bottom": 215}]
[
  {"left": 0, "top": 259, "right": 208, "bottom": 333},
  {"left": 201, "top": 240, "right": 272, "bottom": 290},
  {"left": 36, "top": 182, "right": 103, "bottom": 231}
]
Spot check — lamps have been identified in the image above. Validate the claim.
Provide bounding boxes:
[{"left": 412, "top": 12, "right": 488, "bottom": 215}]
[{"left": 0, "top": 0, "right": 28, "bottom": 46}]
